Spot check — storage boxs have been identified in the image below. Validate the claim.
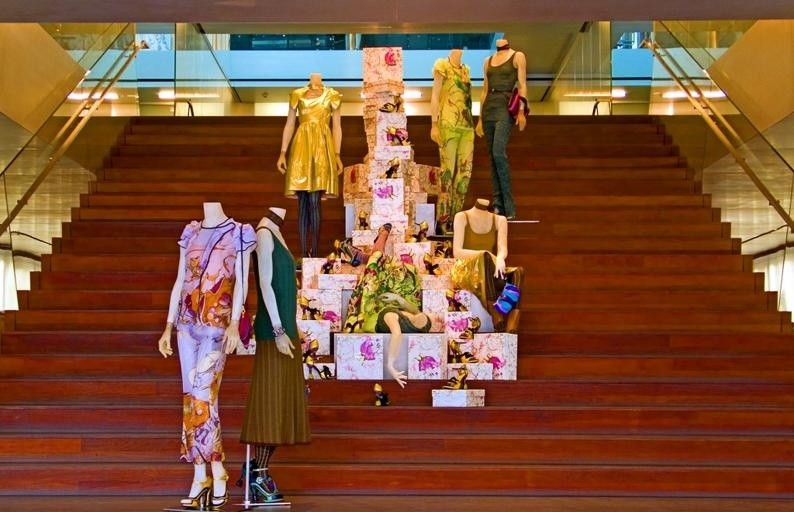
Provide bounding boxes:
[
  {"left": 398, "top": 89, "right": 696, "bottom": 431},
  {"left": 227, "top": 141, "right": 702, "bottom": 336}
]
[
  {"left": 296, "top": 160, "right": 518, "bottom": 380},
  {"left": 431, "top": 389, "right": 485, "bottom": 407},
  {"left": 360, "top": 47, "right": 414, "bottom": 161},
  {"left": 236, "top": 316, "right": 256, "bottom": 355}
]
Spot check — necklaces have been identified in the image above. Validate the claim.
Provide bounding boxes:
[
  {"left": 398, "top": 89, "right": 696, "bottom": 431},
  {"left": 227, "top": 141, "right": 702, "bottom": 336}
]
[{"left": 495, "top": 44, "right": 510, "bottom": 51}]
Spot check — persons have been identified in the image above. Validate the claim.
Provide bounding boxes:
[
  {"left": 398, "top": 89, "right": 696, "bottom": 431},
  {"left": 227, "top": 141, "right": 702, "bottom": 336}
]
[
  {"left": 241, "top": 206, "right": 313, "bottom": 503},
  {"left": 452, "top": 197, "right": 525, "bottom": 331},
  {"left": 475, "top": 39, "right": 528, "bottom": 221},
  {"left": 276, "top": 73, "right": 342, "bottom": 258},
  {"left": 431, "top": 49, "right": 475, "bottom": 224},
  {"left": 157, "top": 200, "right": 257, "bottom": 510},
  {"left": 339, "top": 222, "right": 443, "bottom": 390}
]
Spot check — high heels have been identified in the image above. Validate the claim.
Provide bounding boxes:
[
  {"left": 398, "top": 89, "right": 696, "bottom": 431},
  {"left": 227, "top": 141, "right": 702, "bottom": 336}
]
[
  {"left": 441, "top": 364, "right": 469, "bottom": 390},
  {"left": 305, "top": 355, "right": 337, "bottom": 381},
  {"left": 448, "top": 340, "right": 479, "bottom": 363},
  {"left": 181, "top": 475, "right": 212, "bottom": 508},
  {"left": 335, "top": 239, "right": 360, "bottom": 266},
  {"left": 358, "top": 209, "right": 430, "bottom": 242},
  {"left": 422, "top": 252, "right": 444, "bottom": 276},
  {"left": 235, "top": 457, "right": 256, "bottom": 487},
  {"left": 299, "top": 295, "right": 324, "bottom": 320},
  {"left": 434, "top": 239, "right": 453, "bottom": 259},
  {"left": 320, "top": 252, "right": 336, "bottom": 274},
  {"left": 445, "top": 288, "right": 468, "bottom": 312},
  {"left": 303, "top": 338, "right": 320, "bottom": 362},
  {"left": 249, "top": 473, "right": 284, "bottom": 505}
]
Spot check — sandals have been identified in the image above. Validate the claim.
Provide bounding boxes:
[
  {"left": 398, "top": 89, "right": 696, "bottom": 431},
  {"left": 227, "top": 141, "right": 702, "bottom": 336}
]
[
  {"left": 211, "top": 469, "right": 230, "bottom": 511},
  {"left": 458, "top": 316, "right": 481, "bottom": 340},
  {"left": 373, "top": 381, "right": 391, "bottom": 406}
]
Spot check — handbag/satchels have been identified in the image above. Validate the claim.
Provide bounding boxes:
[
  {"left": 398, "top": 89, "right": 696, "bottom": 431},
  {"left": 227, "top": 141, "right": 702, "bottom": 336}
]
[
  {"left": 239, "top": 310, "right": 251, "bottom": 351},
  {"left": 507, "top": 87, "right": 529, "bottom": 122}
]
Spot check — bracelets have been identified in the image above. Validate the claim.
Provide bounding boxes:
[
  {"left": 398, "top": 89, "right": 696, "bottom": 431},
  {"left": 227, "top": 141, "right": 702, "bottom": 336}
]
[{"left": 272, "top": 326, "right": 286, "bottom": 338}]
